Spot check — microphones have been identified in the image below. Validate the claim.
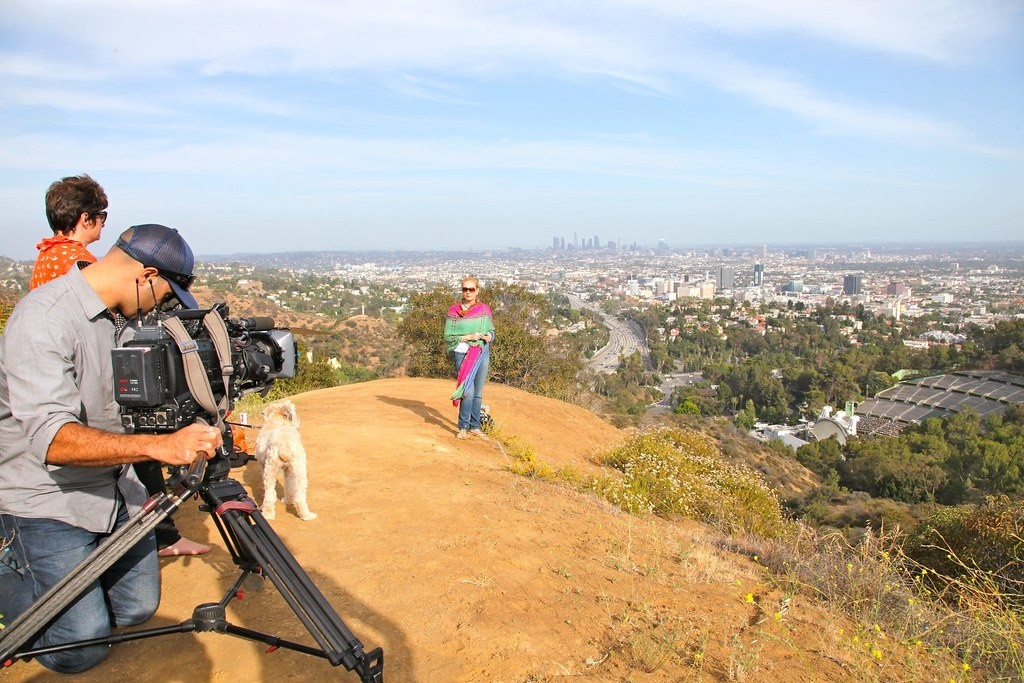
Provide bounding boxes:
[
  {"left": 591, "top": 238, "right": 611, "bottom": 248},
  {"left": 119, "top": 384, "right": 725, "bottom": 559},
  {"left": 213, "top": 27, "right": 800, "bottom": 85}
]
[{"left": 239, "top": 317, "right": 275, "bottom": 332}]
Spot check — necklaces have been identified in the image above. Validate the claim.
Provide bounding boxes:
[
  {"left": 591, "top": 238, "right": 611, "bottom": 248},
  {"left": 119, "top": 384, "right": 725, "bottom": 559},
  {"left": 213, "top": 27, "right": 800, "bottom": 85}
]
[{"left": 463, "top": 298, "right": 469, "bottom": 309}]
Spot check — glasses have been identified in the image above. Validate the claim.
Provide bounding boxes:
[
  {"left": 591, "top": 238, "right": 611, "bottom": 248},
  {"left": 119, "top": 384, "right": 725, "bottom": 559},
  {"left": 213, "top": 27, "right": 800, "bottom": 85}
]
[
  {"left": 144, "top": 264, "right": 197, "bottom": 292},
  {"left": 462, "top": 288, "right": 476, "bottom": 293},
  {"left": 91, "top": 211, "right": 107, "bottom": 223}
]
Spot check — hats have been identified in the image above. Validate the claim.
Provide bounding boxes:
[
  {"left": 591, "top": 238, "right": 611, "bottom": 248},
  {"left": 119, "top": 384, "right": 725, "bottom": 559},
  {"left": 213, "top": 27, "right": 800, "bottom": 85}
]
[{"left": 116, "top": 224, "right": 199, "bottom": 309}]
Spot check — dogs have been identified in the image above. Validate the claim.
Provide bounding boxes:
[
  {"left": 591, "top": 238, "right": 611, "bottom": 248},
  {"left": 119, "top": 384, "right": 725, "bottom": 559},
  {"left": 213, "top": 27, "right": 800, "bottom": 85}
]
[{"left": 253, "top": 398, "right": 317, "bottom": 520}]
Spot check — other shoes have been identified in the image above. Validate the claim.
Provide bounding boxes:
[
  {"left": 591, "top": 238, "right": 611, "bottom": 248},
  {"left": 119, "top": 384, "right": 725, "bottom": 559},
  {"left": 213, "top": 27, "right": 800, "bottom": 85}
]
[
  {"left": 460, "top": 430, "right": 467, "bottom": 439},
  {"left": 469, "top": 428, "right": 490, "bottom": 442}
]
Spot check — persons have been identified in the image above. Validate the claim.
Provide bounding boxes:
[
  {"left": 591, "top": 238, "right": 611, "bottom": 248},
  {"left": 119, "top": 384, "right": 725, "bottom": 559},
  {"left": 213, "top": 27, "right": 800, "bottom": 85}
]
[
  {"left": 444, "top": 277, "right": 495, "bottom": 442},
  {"left": 0, "top": 223, "right": 226, "bottom": 674},
  {"left": 31, "top": 174, "right": 211, "bottom": 556}
]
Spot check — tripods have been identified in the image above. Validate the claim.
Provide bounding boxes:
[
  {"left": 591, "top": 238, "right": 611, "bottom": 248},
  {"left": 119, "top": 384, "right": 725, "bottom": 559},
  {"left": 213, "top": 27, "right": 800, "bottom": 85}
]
[{"left": 0, "top": 406, "right": 386, "bottom": 683}]
